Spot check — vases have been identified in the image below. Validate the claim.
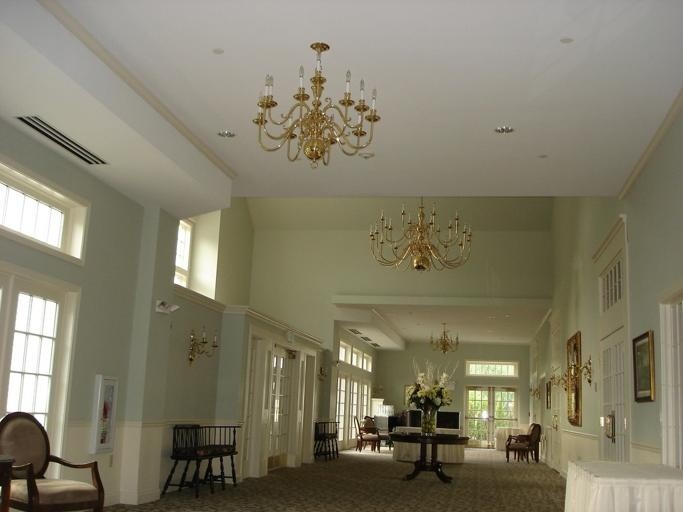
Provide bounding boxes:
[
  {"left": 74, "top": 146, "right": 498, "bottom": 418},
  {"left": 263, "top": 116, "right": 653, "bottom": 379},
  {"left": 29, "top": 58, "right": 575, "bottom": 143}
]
[{"left": 421, "top": 408, "right": 437, "bottom": 433}]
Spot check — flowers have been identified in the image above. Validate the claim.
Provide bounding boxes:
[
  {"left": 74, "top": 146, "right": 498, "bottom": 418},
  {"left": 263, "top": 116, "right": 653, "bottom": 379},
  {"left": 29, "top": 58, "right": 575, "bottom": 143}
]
[{"left": 401, "top": 356, "right": 461, "bottom": 414}]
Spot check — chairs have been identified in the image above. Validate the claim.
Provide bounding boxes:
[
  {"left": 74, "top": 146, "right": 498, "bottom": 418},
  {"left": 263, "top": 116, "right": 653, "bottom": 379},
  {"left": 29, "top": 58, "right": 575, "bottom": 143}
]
[
  {"left": 314, "top": 421, "right": 340, "bottom": 462},
  {"left": 354, "top": 415, "right": 392, "bottom": 453},
  {"left": 505, "top": 423, "right": 542, "bottom": 463},
  {"left": 0, "top": 412, "right": 104, "bottom": 512}
]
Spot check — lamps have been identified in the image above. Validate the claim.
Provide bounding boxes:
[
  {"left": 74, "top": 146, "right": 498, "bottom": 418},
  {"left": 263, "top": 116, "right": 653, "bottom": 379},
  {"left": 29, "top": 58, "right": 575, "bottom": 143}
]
[
  {"left": 368, "top": 197, "right": 472, "bottom": 272},
  {"left": 253, "top": 42, "right": 381, "bottom": 170},
  {"left": 548, "top": 365, "right": 569, "bottom": 391},
  {"left": 567, "top": 359, "right": 592, "bottom": 385},
  {"left": 184, "top": 329, "right": 219, "bottom": 368},
  {"left": 430, "top": 322, "right": 460, "bottom": 355}
]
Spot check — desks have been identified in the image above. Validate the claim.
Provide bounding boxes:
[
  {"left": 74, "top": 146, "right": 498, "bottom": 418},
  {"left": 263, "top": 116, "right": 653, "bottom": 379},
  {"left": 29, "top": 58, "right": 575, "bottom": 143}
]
[
  {"left": 564, "top": 457, "right": 683, "bottom": 511},
  {"left": 389, "top": 431, "right": 469, "bottom": 483}
]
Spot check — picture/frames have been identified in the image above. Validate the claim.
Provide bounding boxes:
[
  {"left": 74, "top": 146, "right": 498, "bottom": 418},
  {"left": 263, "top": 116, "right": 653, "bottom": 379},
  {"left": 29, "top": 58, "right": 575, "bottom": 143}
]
[
  {"left": 632, "top": 328, "right": 655, "bottom": 402},
  {"left": 89, "top": 373, "right": 119, "bottom": 454},
  {"left": 567, "top": 331, "right": 582, "bottom": 426}
]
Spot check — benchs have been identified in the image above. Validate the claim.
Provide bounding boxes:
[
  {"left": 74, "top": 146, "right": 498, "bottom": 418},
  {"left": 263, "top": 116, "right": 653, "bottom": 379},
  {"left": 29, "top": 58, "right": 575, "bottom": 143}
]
[{"left": 161, "top": 423, "right": 242, "bottom": 498}]
